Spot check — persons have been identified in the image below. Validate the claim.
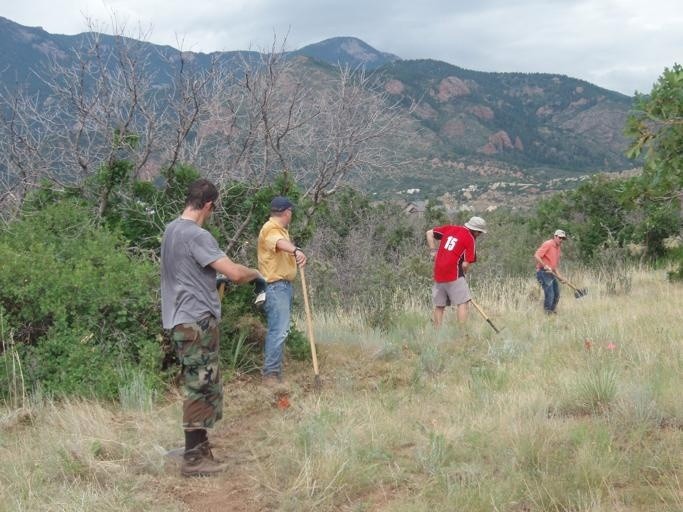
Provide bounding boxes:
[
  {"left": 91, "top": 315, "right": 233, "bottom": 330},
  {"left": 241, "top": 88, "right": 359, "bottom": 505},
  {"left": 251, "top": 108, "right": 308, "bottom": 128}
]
[
  {"left": 534, "top": 229, "right": 568, "bottom": 313},
  {"left": 257, "top": 196, "right": 307, "bottom": 388},
  {"left": 160, "top": 179, "right": 268, "bottom": 478},
  {"left": 426, "top": 216, "right": 487, "bottom": 333}
]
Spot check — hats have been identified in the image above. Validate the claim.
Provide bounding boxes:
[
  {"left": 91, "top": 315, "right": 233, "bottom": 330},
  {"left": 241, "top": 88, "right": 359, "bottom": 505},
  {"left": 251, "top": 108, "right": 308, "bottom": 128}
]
[
  {"left": 554, "top": 229, "right": 567, "bottom": 239},
  {"left": 271, "top": 196, "right": 297, "bottom": 213},
  {"left": 464, "top": 216, "right": 488, "bottom": 234}
]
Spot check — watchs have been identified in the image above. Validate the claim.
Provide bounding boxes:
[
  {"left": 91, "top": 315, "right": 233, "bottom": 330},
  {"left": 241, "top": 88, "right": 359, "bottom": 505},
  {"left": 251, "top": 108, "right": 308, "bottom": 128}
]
[{"left": 294, "top": 246, "right": 303, "bottom": 254}]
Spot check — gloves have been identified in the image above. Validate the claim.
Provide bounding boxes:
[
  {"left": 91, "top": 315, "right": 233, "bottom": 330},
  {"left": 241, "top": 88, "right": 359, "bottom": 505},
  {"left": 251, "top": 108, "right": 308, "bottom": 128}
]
[
  {"left": 249, "top": 269, "right": 268, "bottom": 306},
  {"left": 217, "top": 273, "right": 231, "bottom": 293}
]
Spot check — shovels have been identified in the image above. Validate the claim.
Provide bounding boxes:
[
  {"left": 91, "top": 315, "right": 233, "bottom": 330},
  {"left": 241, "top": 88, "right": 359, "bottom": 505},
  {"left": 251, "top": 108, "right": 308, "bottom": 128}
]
[{"left": 549, "top": 271, "right": 585, "bottom": 298}]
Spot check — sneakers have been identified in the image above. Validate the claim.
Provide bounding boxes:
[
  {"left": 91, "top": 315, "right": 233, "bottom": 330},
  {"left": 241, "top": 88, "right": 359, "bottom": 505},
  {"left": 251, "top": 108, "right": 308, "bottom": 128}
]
[{"left": 181, "top": 439, "right": 231, "bottom": 477}]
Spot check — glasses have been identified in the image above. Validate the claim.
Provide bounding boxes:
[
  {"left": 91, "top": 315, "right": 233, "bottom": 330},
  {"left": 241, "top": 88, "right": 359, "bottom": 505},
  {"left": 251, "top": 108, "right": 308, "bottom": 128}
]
[
  {"left": 559, "top": 236, "right": 566, "bottom": 240},
  {"left": 209, "top": 202, "right": 217, "bottom": 212},
  {"left": 288, "top": 208, "right": 295, "bottom": 212}
]
